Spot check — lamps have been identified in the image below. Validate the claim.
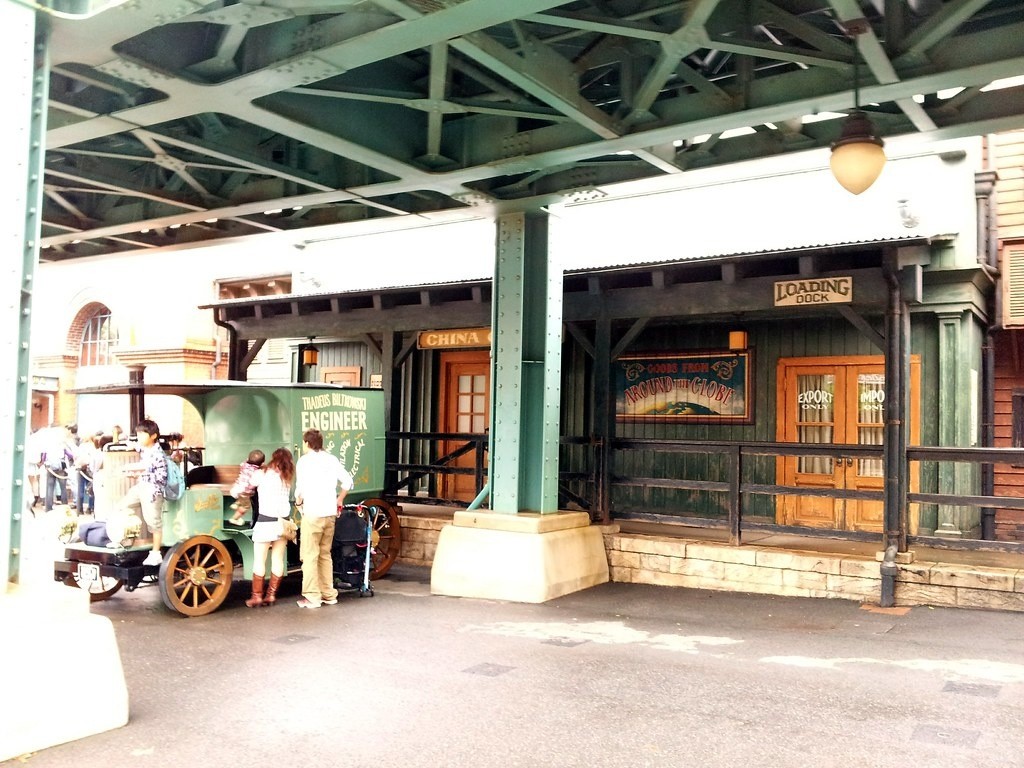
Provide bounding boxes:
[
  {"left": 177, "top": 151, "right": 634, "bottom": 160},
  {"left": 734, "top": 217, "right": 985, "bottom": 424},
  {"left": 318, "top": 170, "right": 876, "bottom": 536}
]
[
  {"left": 828, "top": 19, "right": 884, "bottom": 195},
  {"left": 726, "top": 318, "right": 751, "bottom": 357},
  {"left": 300, "top": 335, "right": 319, "bottom": 370}
]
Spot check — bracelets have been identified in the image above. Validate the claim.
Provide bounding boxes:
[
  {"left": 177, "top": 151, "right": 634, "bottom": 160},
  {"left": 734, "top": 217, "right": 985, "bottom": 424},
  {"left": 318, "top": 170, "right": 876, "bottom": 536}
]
[{"left": 294, "top": 501, "right": 303, "bottom": 507}]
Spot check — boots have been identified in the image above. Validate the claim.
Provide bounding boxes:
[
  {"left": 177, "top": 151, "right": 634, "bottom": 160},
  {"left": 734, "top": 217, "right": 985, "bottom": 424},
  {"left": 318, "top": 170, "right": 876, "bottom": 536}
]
[
  {"left": 263, "top": 572, "right": 284, "bottom": 606},
  {"left": 245, "top": 573, "right": 266, "bottom": 608}
]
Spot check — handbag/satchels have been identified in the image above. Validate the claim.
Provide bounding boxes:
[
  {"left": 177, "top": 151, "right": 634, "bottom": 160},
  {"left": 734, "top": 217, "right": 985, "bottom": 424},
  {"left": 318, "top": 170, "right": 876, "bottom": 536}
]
[{"left": 278, "top": 518, "right": 298, "bottom": 540}]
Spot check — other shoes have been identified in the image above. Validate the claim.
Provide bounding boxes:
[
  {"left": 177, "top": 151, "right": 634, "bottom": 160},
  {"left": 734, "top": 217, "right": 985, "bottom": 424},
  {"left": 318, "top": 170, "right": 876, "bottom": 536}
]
[
  {"left": 71, "top": 505, "right": 77, "bottom": 509},
  {"left": 105, "top": 541, "right": 122, "bottom": 549},
  {"left": 230, "top": 503, "right": 241, "bottom": 510},
  {"left": 76, "top": 512, "right": 84, "bottom": 517},
  {"left": 229, "top": 517, "right": 245, "bottom": 526},
  {"left": 142, "top": 551, "right": 162, "bottom": 566},
  {"left": 85, "top": 508, "right": 91, "bottom": 514}
]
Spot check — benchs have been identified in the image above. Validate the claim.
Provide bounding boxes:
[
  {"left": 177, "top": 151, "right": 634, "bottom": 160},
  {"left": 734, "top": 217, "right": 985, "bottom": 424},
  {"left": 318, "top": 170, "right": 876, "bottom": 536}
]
[{"left": 185, "top": 464, "right": 261, "bottom": 527}]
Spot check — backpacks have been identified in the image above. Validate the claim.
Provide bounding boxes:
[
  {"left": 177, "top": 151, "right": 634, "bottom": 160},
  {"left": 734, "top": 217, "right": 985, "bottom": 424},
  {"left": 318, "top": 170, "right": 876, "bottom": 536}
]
[{"left": 159, "top": 451, "right": 185, "bottom": 502}]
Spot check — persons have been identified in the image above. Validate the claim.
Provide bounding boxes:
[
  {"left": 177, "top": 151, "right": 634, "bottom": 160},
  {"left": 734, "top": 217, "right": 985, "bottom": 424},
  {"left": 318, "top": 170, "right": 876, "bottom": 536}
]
[
  {"left": 229, "top": 449, "right": 266, "bottom": 526},
  {"left": 245, "top": 447, "right": 296, "bottom": 608},
  {"left": 121, "top": 419, "right": 170, "bottom": 566},
  {"left": 164, "top": 423, "right": 200, "bottom": 478},
  {"left": 26, "top": 422, "right": 127, "bottom": 516},
  {"left": 294, "top": 428, "right": 354, "bottom": 609}
]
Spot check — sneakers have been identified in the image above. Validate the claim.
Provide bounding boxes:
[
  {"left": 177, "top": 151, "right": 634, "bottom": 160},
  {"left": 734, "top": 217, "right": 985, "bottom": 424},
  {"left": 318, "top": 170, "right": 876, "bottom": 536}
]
[
  {"left": 296, "top": 598, "right": 321, "bottom": 608},
  {"left": 321, "top": 597, "right": 338, "bottom": 605}
]
